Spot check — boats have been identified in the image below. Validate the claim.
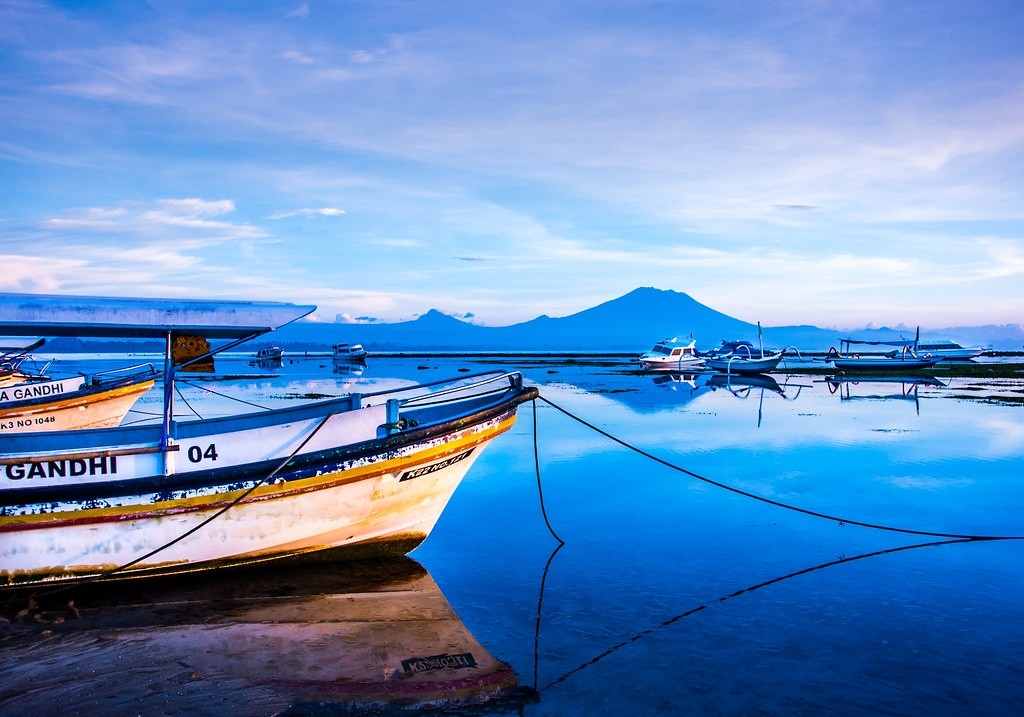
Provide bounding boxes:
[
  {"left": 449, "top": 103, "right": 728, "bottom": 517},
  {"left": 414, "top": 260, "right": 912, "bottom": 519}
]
[
  {"left": 811, "top": 375, "right": 952, "bottom": 417},
  {"left": 249, "top": 345, "right": 285, "bottom": 365},
  {"left": 638, "top": 336, "right": 708, "bottom": 370},
  {"left": 651, "top": 374, "right": 699, "bottom": 392},
  {"left": 708, "top": 322, "right": 788, "bottom": 374},
  {"left": 815, "top": 325, "right": 943, "bottom": 374},
  {"left": 1, "top": 295, "right": 540, "bottom": 598},
  {"left": 908, "top": 337, "right": 987, "bottom": 360},
  {"left": 332, "top": 343, "right": 368, "bottom": 361},
  {"left": 705, "top": 373, "right": 814, "bottom": 429}
]
